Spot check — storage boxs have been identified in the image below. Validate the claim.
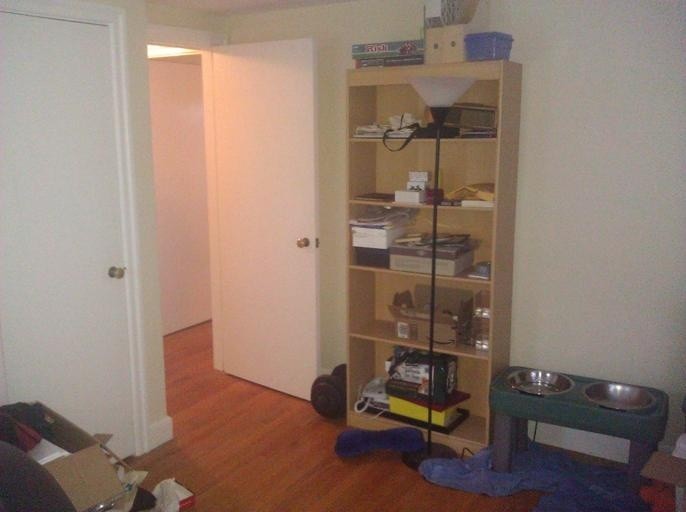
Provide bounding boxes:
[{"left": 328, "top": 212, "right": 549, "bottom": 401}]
[
  {"left": 9, "top": 402, "right": 136, "bottom": 512},
  {"left": 638, "top": 451, "right": 685, "bottom": 512},
  {"left": 388, "top": 284, "right": 482, "bottom": 351},
  {"left": 425, "top": 25, "right": 514, "bottom": 63},
  {"left": 388, "top": 246, "right": 476, "bottom": 278}
]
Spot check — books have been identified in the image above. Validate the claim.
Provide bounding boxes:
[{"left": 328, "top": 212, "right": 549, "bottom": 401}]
[{"left": 350, "top": 100, "right": 497, "bottom": 255}]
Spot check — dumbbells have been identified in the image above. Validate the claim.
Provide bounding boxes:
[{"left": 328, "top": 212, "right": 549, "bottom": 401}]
[{"left": 311, "top": 363, "right": 346, "bottom": 419}]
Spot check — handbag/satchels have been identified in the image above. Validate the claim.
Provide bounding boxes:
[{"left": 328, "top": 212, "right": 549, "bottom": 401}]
[{"left": 383, "top": 123, "right": 460, "bottom": 153}]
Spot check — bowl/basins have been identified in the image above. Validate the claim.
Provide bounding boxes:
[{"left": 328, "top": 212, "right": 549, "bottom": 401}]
[{"left": 505, "top": 369, "right": 655, "bottom": 411}]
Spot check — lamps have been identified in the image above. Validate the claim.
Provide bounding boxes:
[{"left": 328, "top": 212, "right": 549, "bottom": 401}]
[{"left": 401, "top": 74, "right": 474, "bottom": 472}]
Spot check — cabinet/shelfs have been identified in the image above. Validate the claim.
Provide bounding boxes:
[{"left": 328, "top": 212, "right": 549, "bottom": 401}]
[{"left": 343, "top": 60, "right": 523, "bottom": 458}]
